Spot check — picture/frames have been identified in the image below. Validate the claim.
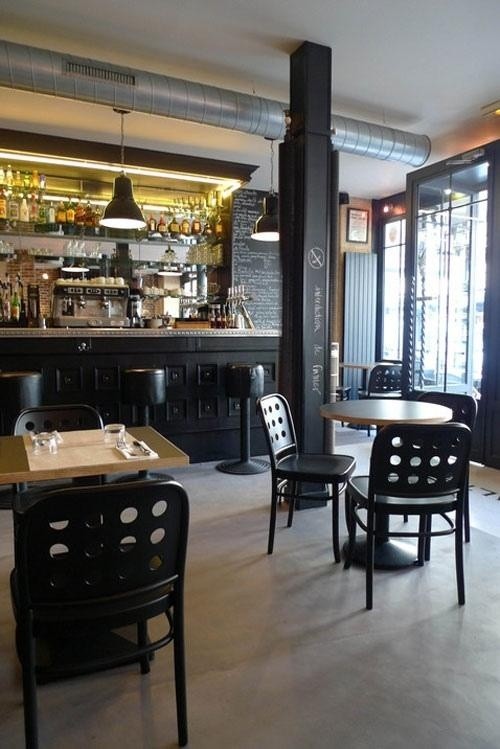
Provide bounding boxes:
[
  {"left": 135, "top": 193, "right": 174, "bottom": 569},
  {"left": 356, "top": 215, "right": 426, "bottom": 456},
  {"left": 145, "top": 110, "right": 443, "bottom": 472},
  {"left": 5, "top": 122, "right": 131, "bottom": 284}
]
[{"left": 346, "top": 207, "right": 369, "bottom": 244}]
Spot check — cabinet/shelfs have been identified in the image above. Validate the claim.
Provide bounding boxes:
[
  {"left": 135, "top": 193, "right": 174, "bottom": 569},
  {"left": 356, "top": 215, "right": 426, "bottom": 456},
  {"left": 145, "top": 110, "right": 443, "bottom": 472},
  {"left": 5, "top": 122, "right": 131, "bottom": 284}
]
[{"left": 0, "top": 327, "right": 283, "bottom": 462}]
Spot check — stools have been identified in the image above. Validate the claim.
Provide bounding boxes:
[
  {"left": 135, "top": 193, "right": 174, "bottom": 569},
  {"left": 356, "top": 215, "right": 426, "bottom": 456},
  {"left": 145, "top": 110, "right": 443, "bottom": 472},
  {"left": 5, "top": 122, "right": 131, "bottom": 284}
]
[
  {"left": 336, "top": 386, "right": 351, "bottom": 426},
  {"left": 0, "top": 372, "right": 42, "bottom": 510},
  {"left": 215, "top": 362, "right": 270, "bottom": 475},
  {"left": 116, "top": 368, "right": 175, "bottom": 483}
]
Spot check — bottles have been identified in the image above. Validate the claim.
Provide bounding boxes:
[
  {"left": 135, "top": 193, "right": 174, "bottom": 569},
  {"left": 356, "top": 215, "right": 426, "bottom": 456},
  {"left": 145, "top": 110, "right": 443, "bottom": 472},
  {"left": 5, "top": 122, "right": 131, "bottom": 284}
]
[
  {"left": 6, "top": 165, "right": 14, "bottom": 185},
  {"left": 40, "top": 174, "right": 46, "bottom": 189},
  {"left": 217, "top": 305, "right": 222, "bottom": 328},
  {"left": 148, "top": 212, "right": 157, "bottom": 231},
  {"left": 76, "top": 205, "right": 85, "bottom": 224},
  {"left": 66, "top": 203, "right": 75, "bottom": 224},
  {"left": 30, "top": 191, "right": 37, "bottom": 222},
  {"left": 24, "top": 171, "right": 30, "bottom": 188},
  {"left": 157, "top": 211, "right": 166, "bottom": 232},
  {"left": 210, "top": 306, "right": 217, "bottom": 328},
  {"left": 20, "top": 199, "right": 29, "bottom": 221},
  {"left": 168, "top": 218, "right": 181, "bottom": 234},
  {"left": 192, "top": 217, "right": 201, "bottom": 234},
  {"left": 86, "top": 201, "right": 93, "bottom": 225},
  {"left": 8, "top": 195, "right": 20, "bottom": 222},
  {"left": 48, "top": 202, "right": 55, "bottom": 223},
  {"left": 0, "top": 191, "right": 8, "bottom": 219},
  {"left": 95, "top": 205, "right": 101, "bottom": 225},
  {"left": 214, "top": 207, "right": 223, "bottom": 236},
  {"left": 15, "top": 171, "right": 21, "bottom": 186},
  {"left": 0, "top": 167, "right": 6, "bottom": 184},
  {"left": 11, "top": 292, "right": 19, "bottom": 321},
  {"left": 221, "top": 304, "right": 227, "bottom": 329},
  {"left": 14, "top": 189, "right": 20, "bottom": 200},
  {"left": 39, "top": 192, "right": 46, "bottom": 224},
  {"left": 3, "top": 293, "right": 10, "bottom": 321},
  {"left": 32, "top": 170, "right": 39, "bottom": 188},
  {"left": 203, "top": 216, "right": 213, "bottom": 235},
  {"left": 57, "top": 201, "right": 66, "bottom": 223}
]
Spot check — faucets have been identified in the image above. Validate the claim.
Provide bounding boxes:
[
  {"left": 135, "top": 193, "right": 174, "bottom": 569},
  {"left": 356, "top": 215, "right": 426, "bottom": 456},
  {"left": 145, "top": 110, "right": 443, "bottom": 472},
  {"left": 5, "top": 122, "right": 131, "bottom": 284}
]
[
  {"left": 78, "top": 296, "right": 86, "bottom": 308},
  {"left": 100, "top": 296, "right": 109, "bottom": 309}
]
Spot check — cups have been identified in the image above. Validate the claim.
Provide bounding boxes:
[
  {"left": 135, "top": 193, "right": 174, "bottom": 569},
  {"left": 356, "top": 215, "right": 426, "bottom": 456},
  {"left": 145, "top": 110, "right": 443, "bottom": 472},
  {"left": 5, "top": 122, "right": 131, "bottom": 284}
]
[
  {"left": 105, "top": 424, "right": 125, "bottom": 450},
  {"left": 34, "top": 433, "right": 59, "bottom": 457}
]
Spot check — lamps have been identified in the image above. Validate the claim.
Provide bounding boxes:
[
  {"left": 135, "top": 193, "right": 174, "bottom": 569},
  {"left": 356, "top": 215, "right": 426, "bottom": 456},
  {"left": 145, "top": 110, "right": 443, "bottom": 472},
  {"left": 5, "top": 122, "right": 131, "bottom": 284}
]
[
  {"left": 250, "top": 140, "right": 280, "bottom": 242},
  {"left": 99, "top": 108, "right": 147, "bottom": 230}
]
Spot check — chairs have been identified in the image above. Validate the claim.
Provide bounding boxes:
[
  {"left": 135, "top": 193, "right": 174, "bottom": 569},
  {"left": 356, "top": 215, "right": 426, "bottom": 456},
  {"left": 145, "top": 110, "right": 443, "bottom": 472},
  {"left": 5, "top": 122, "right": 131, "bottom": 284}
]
[
  {"left": 402, "top": 392, "right": 478, "bottom": 544},
  {"left": 342, "top": 422, "right": 473, "bottom": 610},
  {"left": 257, "top": 393, "right": 357, "bottom": 563},
  {"left": 13, "top": 404, "right": 104, "bottom": 435},
  {"left": 358, "top": 364, "right": 403, "bottom": 436},
  {"left": 8, "top": 480, "right": 190, "bottom": 749}
]
